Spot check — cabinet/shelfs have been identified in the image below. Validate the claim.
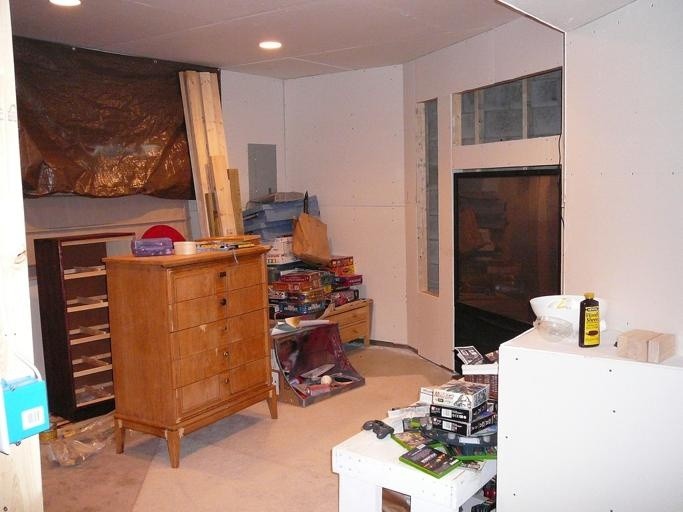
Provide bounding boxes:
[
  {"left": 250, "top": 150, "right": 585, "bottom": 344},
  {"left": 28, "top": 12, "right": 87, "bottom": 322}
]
[
  {"left": 35, "top": 237, "right": 145, "bottom": 425},
  {"left": 95, "top": 244, "right": 279, "bottom": 468},
  {"left": 495, "top": 328, "right": 683, "bottom": 512}
]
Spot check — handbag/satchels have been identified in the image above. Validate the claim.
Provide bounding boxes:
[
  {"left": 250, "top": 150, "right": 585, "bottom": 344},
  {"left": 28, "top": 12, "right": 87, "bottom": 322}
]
[{"left": 292, "top": 212, "right": 329, "bottom": 266}]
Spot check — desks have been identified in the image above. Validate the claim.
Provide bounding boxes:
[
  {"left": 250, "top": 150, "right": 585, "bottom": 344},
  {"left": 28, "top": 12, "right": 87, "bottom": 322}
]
[
  {"left": 320, "top": 299, "right": 373, "bottom": 346},
  {"left": 329, "top": 414, "right": 496, "bottom": 511}
]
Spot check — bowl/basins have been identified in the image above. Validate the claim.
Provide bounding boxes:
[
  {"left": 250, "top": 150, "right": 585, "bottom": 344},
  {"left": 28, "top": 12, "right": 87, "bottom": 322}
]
[
  {"left": 530, "top": 294, "right": 609, "bottom": 334},
  {"left": 533, "top": 316, "right": 574, "bottom": 342}
]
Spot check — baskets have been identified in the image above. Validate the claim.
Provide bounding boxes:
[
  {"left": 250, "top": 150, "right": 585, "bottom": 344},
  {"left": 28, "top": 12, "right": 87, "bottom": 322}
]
[{"left": 461, "top": 363, "right": 498, "bottom": 402}]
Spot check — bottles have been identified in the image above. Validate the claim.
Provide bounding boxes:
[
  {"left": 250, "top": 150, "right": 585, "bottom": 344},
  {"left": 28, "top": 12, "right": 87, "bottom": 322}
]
[{"left": 579, "top": 292, "right": 601, "bottom": 347}]
